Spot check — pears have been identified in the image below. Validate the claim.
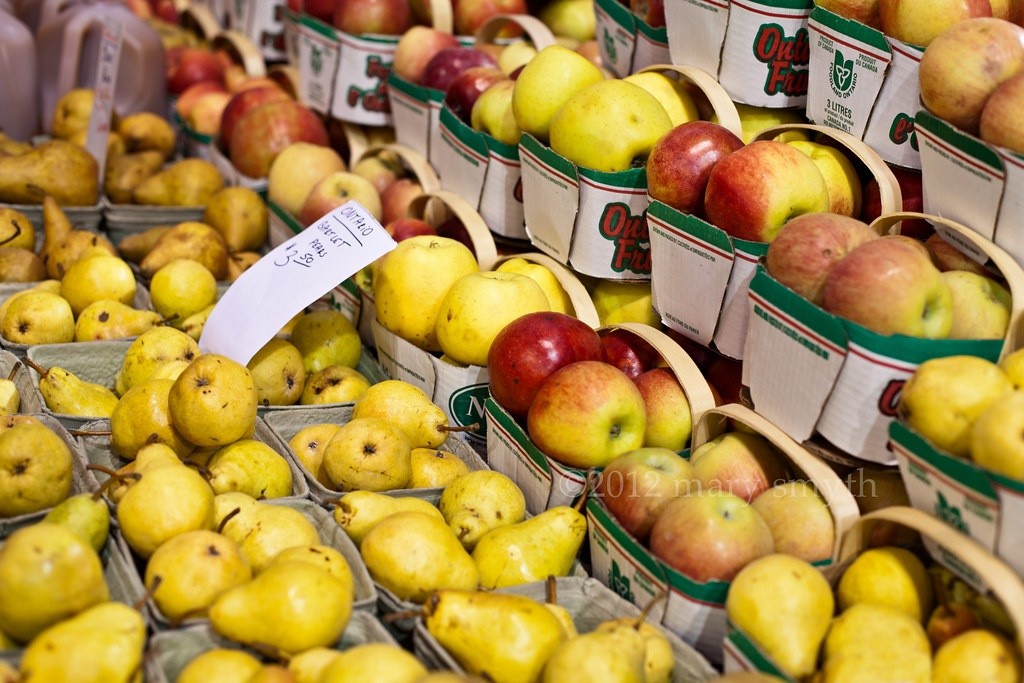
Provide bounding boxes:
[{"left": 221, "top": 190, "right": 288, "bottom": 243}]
[{"left": 0, "top": 87, "right": 1023, "bottom": 682}]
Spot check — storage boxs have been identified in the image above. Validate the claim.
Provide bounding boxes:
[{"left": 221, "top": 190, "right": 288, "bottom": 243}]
[{"left": 1, "top": 0, "right": 1024, "bottom": 683}]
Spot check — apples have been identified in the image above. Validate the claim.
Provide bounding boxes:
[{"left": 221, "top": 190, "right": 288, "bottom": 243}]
[{"left": 130, "top": 0, "right": 1024, "bottom": 587}]
